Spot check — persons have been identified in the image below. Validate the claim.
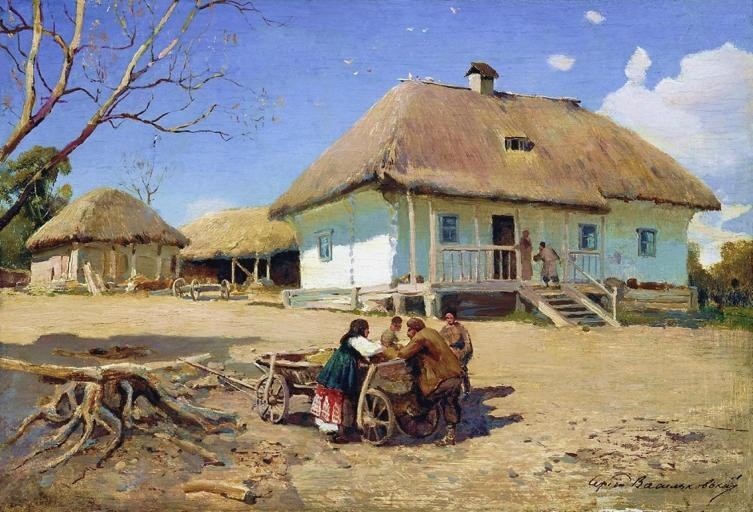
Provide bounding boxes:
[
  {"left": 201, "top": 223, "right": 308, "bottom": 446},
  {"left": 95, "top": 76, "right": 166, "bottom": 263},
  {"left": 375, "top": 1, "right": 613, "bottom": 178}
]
[
  {"left": 309, "top": 318, "right": 399, "bottom": 445},
  {"left": 380, "top": 314, "right": 403, "bottom": 349},
  {"left": 438, "top": 308, "right": 474, "bottom": 402},
  {"left": 395, "top": 317, "right": 466, "bottom": 447},
  {"left": 532, "top": 241, "right": 561, "bottom": 289},
  {"left": 517, "top": 229, "right": 535, "bottom": 281}
]
